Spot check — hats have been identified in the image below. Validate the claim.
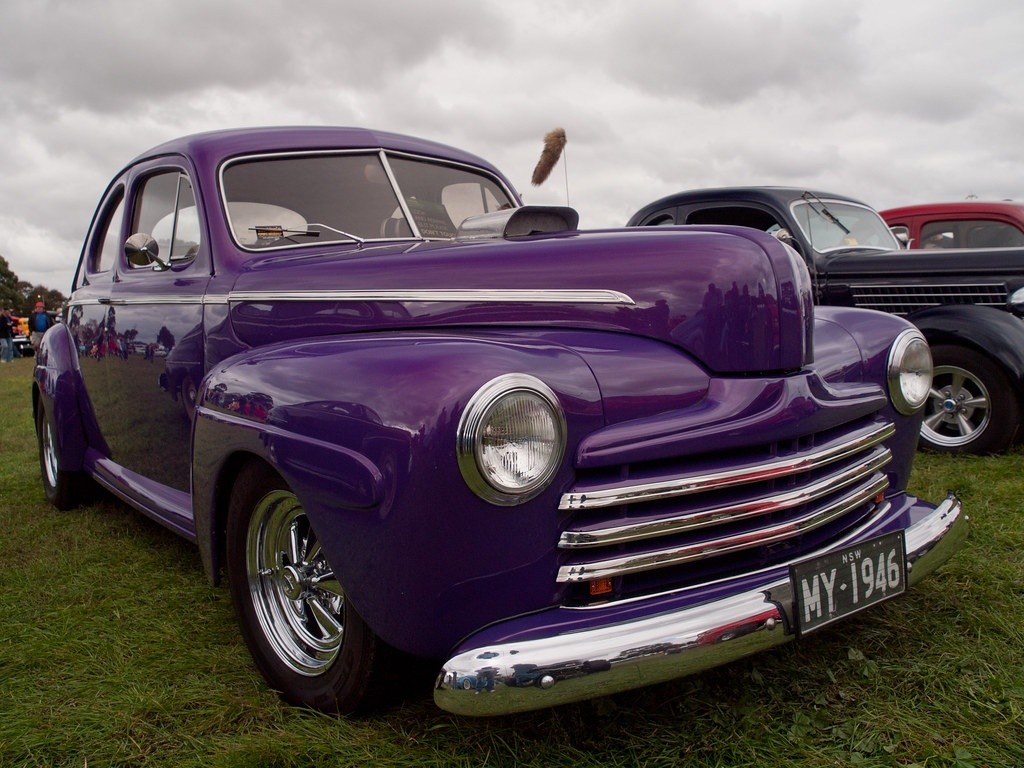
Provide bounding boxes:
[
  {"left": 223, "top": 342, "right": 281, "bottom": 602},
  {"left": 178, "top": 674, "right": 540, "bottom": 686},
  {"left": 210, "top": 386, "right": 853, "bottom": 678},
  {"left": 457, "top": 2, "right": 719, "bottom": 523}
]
[{"left": 34, "top": 302, "right": 46, "bottom": 309}]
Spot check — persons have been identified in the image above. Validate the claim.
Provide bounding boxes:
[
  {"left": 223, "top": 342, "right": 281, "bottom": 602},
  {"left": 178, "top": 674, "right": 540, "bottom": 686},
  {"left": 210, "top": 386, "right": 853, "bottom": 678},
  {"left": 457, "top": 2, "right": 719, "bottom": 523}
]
[
  {"left": 0, "top": 305, "right": 15, "bottom": 363},
  {"left": 930, "top": 233, "right": 954, "bottom": 248},
  {"left": 74, "top": 333, "right": 156, "bottom": 361},
  {"left": 206, "top": 386, "right": 241, "bottom": 412},
  {"left": 28, "top": 301, "right": 54, "bottom": 359}
]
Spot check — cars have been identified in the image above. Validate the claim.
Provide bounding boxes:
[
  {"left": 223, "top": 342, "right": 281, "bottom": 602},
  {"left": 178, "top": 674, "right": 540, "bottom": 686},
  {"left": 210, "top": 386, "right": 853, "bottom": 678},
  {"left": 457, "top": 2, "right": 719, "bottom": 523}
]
[
  {"left": 831, "top": 201, "right": 1024, "bottom": 248},
  {"left": 624, "top": 184, "right": 1024, "bottom": 456},
  {"left": 31, "top": 124, "right": 966, "bottom": 719}
]
